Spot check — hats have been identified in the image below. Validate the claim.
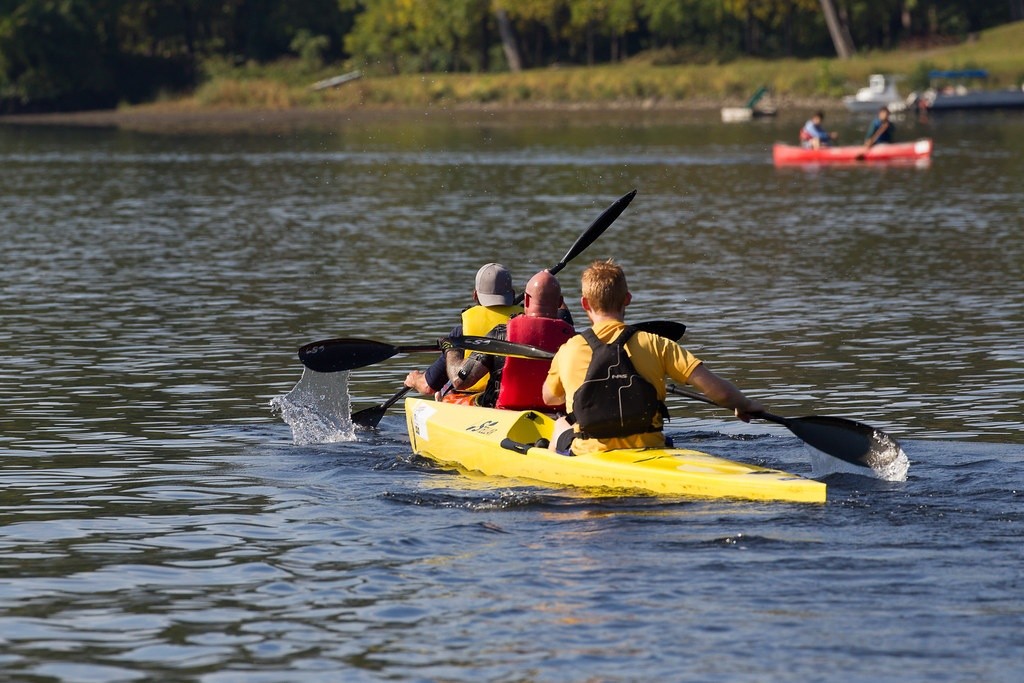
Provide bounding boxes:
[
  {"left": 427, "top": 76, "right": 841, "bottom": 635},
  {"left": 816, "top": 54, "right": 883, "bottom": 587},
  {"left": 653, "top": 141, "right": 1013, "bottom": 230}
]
[{"left": 475, "top": 262, "right": 514, "bottom": 308}]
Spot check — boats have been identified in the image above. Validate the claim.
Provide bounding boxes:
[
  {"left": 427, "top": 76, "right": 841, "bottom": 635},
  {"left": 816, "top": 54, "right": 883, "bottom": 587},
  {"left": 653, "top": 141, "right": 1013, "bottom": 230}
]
[
  {"left": 841, "top": 74, "right": 903, "bottom": 116},
  {"left": 921, "top": 69, "right": 1023, "bottom": 111},
  {"left": 770, "top": 139, "right": 932, "bottom": 168},
  {"left": 403, "top": 389, "right": 829, "bottom": 505}
]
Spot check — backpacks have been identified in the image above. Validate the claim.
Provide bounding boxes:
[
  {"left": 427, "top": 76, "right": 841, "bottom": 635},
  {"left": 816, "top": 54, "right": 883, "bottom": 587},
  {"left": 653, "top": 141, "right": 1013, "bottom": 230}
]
[{"left": 571, "top": 325, "right": 671, "bottom": 439}]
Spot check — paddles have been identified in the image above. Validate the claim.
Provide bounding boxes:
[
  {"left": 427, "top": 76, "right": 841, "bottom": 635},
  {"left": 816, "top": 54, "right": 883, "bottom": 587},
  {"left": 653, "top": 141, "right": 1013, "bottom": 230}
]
[
  {"left": 441, "top": 333, "right": 901, "bottom": 470},
  {"left": 345, "top": 188, "right": 643, "bottom": 427},
  {"left": 853, "top": 119, "right": 891, "bottom": 161},
  {"left": 294, "top": 320, "right": 690, "bottom": 372}
]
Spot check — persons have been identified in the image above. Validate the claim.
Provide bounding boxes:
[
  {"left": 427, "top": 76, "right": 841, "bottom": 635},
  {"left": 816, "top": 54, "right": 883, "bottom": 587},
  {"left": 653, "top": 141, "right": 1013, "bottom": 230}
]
[
  {"left": 403, "top": 262, "right": 574, "bottom": 407},
  {"left": 433, "top": 269, "right": 576, "bottom": 411},
  {"left": 866, "top": 107, "right": 897, "bottom": 144},
  {"left": 799, "top": 110, "right": 839, "bottom": 149},
  {"left": 542, "top": 263, "right": 766, "bottom": 456}
]
